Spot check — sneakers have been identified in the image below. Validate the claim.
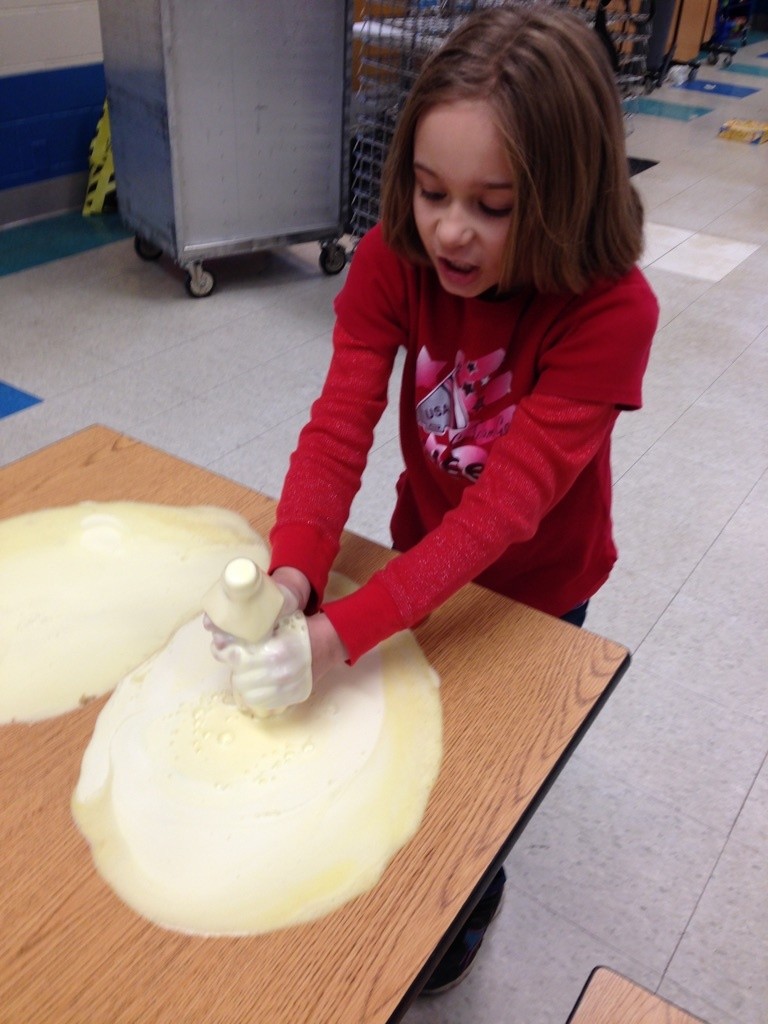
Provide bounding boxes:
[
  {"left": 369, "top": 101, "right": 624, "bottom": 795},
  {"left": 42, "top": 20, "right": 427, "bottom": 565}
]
[{"left": 422, "top": 868, "right": 510, "bottom": 997}]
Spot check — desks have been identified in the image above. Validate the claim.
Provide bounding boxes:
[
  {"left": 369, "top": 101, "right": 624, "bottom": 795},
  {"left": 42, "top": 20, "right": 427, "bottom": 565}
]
[{"left": 1, "top": 421, "right": 634, "bottom": 1022}]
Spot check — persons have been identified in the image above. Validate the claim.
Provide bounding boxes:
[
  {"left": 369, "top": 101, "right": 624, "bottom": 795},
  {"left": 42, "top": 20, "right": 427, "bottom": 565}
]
[{"left": 203, "top": 1, "right": 662, "bottom": 999}]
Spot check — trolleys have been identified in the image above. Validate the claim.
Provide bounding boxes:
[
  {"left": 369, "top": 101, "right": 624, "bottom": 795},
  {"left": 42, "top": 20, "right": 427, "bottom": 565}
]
[{"left": 99, "top": 0, "right": 353, "bottom": 301}]
